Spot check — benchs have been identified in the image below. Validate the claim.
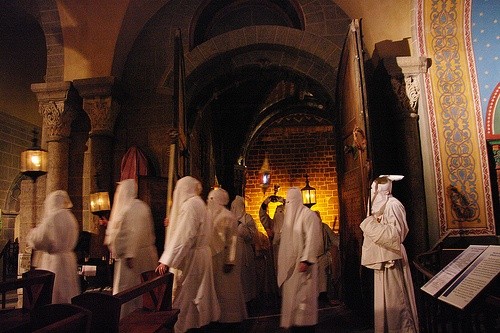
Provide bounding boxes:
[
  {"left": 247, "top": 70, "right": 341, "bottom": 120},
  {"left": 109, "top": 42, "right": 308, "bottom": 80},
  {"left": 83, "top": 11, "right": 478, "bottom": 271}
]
[
  {"left": 0, "top": 302, "right": 93, "bottom": 333},
  {"left": 71, "top": 269, "right": 180, "bottom": 333}
]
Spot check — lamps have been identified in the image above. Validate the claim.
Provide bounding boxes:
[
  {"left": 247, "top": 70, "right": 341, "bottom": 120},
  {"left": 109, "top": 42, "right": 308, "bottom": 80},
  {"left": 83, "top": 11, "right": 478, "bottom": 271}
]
[
  {"left": 22, "top": 126, "right": 49, "bottom": 229},
  {"left": 262, "top": 132, "right": 272, "bottom": 198},
  {"left": 301, "top": 173, "right": 316, "bottom": 212},
  {"left": 89, "top": 172, "right": 111, "bottom": 229}
]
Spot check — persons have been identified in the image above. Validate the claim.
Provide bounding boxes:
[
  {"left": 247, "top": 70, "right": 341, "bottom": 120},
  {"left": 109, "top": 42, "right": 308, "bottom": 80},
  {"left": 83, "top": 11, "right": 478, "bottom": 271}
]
[
  {"left": 360, "top": 177, "right": 419, "bottom": 333},
  {"left": 103, "top": 178, "right": 159, "bottom": 321},
  {"left": 26, "top": 190, "right": 81, "bottom": 305},
  {"left": 155, "top": 176, "right": 339, "bottom": 333}
]
